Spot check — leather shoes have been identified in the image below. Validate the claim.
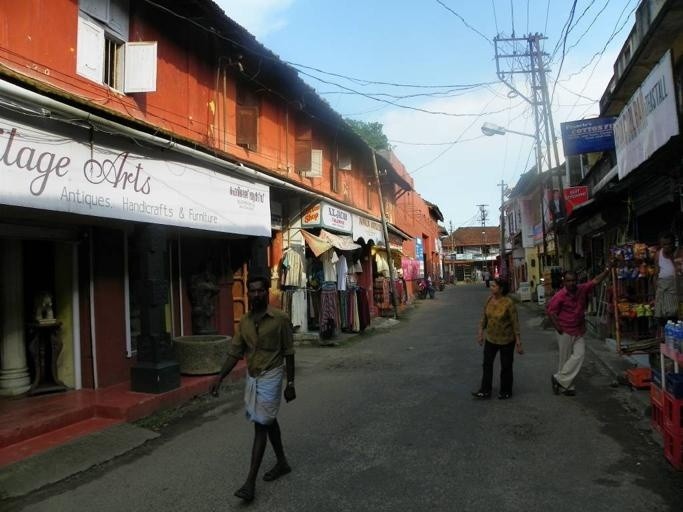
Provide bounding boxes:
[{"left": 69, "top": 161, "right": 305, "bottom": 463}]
[
  {"left": 550, "top": 375, "right": 561, "bottom": 395},
  {"left": 497, "top": 392, "right": 512, "bottom": 399},
  {"left": 472, "top": 391, "right": 491, "bottom": 398},
  {"left": 563, "top": 390, "right": 574, "bottom": 396}
]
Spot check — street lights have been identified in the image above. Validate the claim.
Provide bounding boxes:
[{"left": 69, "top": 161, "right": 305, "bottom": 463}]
[
  {"left": 479, "top": 117, "right": 556, "bottom": 264},
  {"left": 481, "top": 230, "right": 485, "bottom": 271}
]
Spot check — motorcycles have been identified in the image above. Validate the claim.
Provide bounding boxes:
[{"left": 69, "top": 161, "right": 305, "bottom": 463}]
[
  {"left": 437, "top": 276, "right": 446, "bottom": 292},
  {"left": 415, "top": 279, "right": 428, "bottom": 299}
]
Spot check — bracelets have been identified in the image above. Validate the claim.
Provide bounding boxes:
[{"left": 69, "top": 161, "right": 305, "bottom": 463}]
[
  {"left": 605, "top": 267, "right": 609, "bottom": 271},
  {"left": 286, "top": 380, "right": 294, "bottom": 388}
]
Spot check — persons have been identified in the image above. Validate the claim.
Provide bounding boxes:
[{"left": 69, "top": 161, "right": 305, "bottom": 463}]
[
  {"left": 481, "top": 269, "right": 491, "bottom": 288},
  {"left": 427, "top": 271, "right": 432, "bottom": 285},
  {"left": 542, "top": 257, "right": 617, "bottom": 399},
  {"left": 651, "top": 230, "right": 682, "bottom": 327},
  {"left": 549, "top": 190, "right": 561, "bottom": 220},
  {"left": 207, "top": 274, "right": 296, "bottom": 502},
  {"left": 469, "top": 277, "right": 525, "bottom": 400}
]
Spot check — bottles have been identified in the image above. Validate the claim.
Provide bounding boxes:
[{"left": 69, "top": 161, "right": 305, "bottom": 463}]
[{"left": 663, "top": 318, "right": 683, "bottom": 357}]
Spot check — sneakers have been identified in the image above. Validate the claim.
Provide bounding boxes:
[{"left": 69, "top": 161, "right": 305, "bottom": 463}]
[
  {"left": 262, "top": 466, "right": 290, "bottom": 481},
  {"left": 234, "top": 487, "right": 254, "bottom": 502}
]
